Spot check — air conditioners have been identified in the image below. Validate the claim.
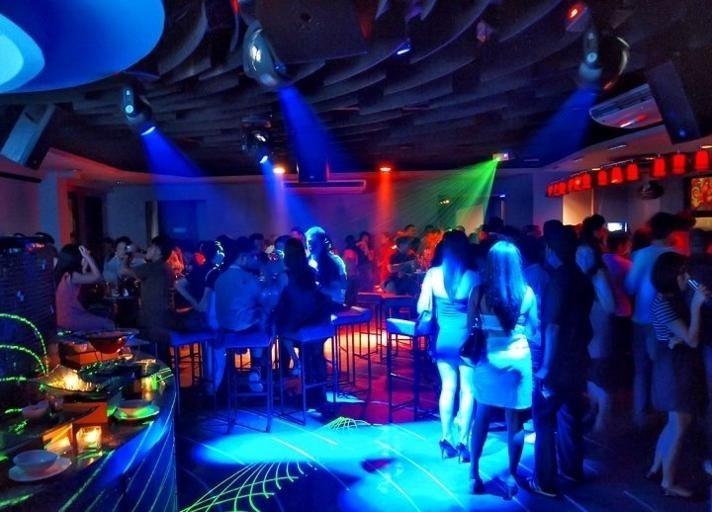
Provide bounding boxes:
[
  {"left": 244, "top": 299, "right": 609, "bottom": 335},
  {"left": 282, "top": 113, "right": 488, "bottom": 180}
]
[{"left": 588, "top": 84, "right": 662, "bottom": 131}]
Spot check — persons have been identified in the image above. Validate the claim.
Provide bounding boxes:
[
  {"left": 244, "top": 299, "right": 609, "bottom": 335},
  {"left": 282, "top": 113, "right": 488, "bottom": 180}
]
[
  {"left": 414, "top": 217, "right": 712, "bottom": 497},
  {"left": 0, "top": 224, "right": 416, "bottom": 400}
]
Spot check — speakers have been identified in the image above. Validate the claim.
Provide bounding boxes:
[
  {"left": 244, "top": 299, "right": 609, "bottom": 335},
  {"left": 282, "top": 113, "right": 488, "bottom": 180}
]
[
  {"left": 2, "top": 103, "right": 70, "bottom": 170},
  {"left": 644, "top": 51, "right": 712, "bottom": 145}
]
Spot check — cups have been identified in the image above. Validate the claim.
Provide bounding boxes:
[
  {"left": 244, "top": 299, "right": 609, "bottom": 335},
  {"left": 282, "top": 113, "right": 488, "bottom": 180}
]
[
  {"left": 76, "top": 426, "right": 102, "bottom": 455},
  {"left": 687, "top": 277, "right": 712, "bottom": 307}
]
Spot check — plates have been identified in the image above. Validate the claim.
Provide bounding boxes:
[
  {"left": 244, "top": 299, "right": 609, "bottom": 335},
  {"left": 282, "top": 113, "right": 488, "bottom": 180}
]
[
  {"left": 113, "top": 404, "right": 161, "bottom": 421},
  {"left": 9, "top": 456, "right": 72, "bottom": 481}
]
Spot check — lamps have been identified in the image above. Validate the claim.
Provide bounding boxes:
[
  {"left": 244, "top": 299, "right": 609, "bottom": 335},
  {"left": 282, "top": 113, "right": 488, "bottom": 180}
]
[
  {"left": 571, "top": 16, "right": 632, "bottom": 92},
  {"left": 240, "top": 116, "right": 274, "bottom": 165},
  {"left": 123, "top": 89, "right": 156, "bottom": 138}
]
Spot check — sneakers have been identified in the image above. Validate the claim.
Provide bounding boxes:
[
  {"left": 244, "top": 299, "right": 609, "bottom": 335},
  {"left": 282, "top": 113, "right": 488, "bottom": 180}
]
[
  {"left": 292, "top": 362, "right": 301, "bottom": 376},
  {"left": 526, "top": 476, "right": 556, "bottom": 496},
  {"left": 660, "top": 482, "right": 692, "bottom": 497},
  {"left": 248, "top": 370, "right": 263, "bottom": 392}
]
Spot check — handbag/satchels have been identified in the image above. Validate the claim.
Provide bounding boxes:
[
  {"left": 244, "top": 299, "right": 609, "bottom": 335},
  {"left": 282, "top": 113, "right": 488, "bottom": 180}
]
[
  {"left": 459, "top": 318, "right": 481, "bottom": 367},
  {"left": 414, "top": 310, "right": 436, "bottom": 337}
]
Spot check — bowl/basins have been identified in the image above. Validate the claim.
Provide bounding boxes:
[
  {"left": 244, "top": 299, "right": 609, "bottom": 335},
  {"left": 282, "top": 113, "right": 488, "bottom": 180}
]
[
  {"left": 116, "top": 399, "right": 148, "bottom": 417},
  {"left": 12, "top": 450, "right": 59, "bottom": 475}
]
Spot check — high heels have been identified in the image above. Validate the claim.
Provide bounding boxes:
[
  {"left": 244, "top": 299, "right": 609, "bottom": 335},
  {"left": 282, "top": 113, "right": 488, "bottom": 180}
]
[
  {"left": 440, "top": 438, "right": 457, "bottom": 459},
  {"left": 456, "top": 443, "right": 470, "bottom": 464},
  {"left": 504, "top": 482, "right": 518, "bottom": 499},
  {"left": 470, "top": 479, "right": 482, "bottom": 494}
]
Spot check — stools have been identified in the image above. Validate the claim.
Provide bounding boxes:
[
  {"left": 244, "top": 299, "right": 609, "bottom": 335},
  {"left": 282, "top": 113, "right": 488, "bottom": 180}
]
[{"left": 151, "top": 291, "right": 438, "bottom": 435}]
